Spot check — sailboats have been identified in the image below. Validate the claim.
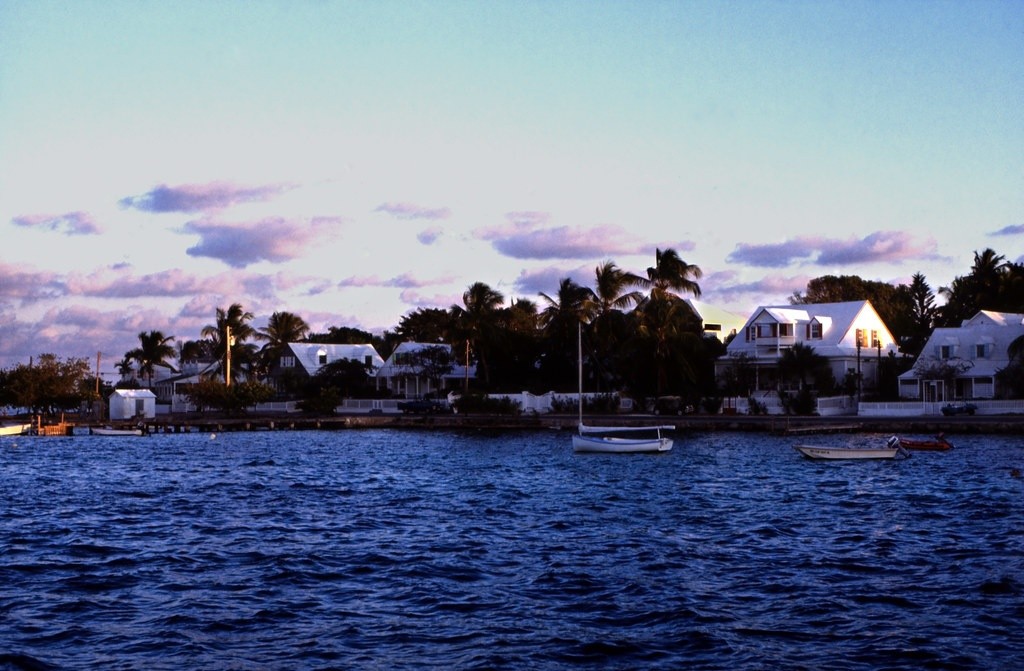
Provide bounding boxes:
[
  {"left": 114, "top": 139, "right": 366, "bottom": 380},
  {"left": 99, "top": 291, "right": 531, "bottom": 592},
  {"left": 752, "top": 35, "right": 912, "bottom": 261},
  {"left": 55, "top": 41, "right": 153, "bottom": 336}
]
[{"left": 570, "top": 321, "right": 678, "bottom": 453}]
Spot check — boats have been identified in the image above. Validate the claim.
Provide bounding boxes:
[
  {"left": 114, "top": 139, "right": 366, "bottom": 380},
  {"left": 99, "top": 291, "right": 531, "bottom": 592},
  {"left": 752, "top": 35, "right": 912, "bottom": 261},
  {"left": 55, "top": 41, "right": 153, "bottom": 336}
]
[
  {"left": 898, "top": 430, "right": 958, "bottom": 452},
  {"left": 791, "top": 434, "right": 912, "bottom": 461},
  {"left": 87, "top": 425, "right": 143, "bottom": 437}
]
